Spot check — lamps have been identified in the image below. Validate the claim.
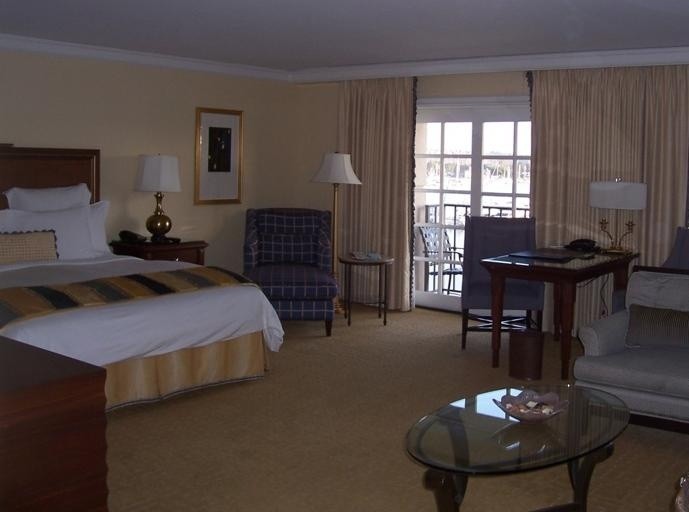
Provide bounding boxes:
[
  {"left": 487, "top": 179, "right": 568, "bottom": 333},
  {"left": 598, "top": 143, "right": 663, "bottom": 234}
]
[
  {"left": 130, "top": 149, "right": 183, "bottom": 241},
  {"left": 586, "top": 176, "right": 650, "bottom": 256},
  {"left": 306, "top": 150, "right": 364, "bottom": 315}
]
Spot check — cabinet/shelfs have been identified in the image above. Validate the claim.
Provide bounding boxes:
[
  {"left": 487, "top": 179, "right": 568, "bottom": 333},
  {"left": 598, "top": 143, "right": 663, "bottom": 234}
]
[{"left": 0, "top": 334, "right": 109, "bottom": 512}]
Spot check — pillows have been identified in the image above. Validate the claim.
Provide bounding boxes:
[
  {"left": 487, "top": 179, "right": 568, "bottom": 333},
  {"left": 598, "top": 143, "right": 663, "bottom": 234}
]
[
  {"left": 1, "top": 184, "right": 110, "bottom": 266},
  {"left": 623, "top": 302, "right": 689, "bottom": 350}
]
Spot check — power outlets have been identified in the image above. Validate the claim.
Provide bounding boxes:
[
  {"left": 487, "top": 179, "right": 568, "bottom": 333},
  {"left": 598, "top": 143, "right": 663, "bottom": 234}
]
[{"left": 598, "top": 305, "right": 609, "bottom": 316}]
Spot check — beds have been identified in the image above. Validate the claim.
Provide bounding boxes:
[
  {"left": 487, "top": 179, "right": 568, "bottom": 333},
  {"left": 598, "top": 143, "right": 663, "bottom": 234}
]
[{"left": 0, "top": 142, "right": 287, "bottom": 415}]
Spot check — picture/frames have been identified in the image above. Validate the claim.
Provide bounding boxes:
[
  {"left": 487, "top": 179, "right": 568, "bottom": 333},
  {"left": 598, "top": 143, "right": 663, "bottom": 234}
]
[{"left": 194, "top": 106, "right": 245, "bottom": 208}]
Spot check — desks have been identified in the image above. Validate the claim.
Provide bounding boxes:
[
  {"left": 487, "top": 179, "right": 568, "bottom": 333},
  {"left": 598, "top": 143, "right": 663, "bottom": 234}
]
[
  {"left": 479, "top": 243, "right": 642, "bottom": 381},
  {"left": 400, "top": 383, "right": 631, "bottom": 512},
  {"left": 336, "top": 253, "right": 395, "bottom": 328}
]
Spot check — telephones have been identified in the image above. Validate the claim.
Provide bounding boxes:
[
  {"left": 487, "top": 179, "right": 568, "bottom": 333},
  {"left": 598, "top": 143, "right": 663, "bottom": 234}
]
[
  {"left": 569, "top": 239, "right": 601, "bottom": 251},
  {"left": 119, "top": 230, "right": 147, "bottom": 244}
]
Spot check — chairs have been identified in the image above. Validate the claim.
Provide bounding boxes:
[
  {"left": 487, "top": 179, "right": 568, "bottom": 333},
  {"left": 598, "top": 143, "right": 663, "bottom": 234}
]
[
  {"left": 243, "top": 206, "right": 340, "bottom": 339},
  {"left": 460, "top": 213, "right": 545, "bottom": 351},
  {"left": 416, "top": 222, "right": 467, "bottom": 296}
]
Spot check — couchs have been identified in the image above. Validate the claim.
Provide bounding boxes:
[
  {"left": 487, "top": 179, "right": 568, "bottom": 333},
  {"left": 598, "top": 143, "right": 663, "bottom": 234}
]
[{"left": 574, "top": 269, "right": 688, "bottom": 427}]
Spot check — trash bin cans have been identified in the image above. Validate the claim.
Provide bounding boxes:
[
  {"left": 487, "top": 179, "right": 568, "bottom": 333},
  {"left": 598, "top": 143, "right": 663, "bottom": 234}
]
[{"left": 509, "top": 329, "right": 543, "bottom": 381}]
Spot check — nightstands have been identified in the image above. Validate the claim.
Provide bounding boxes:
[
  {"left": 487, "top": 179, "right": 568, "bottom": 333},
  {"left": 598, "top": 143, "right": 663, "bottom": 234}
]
[{"left": 105, "top": 237, "right": 209, "bottom": 265}]
